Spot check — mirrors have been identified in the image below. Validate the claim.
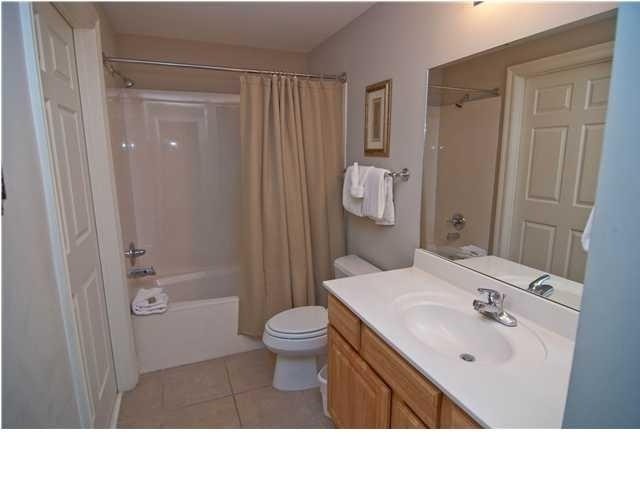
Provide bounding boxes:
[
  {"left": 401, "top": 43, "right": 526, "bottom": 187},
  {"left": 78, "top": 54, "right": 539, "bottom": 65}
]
[{"left": 418, "top": 8, "right": 617, "bottom": 312}]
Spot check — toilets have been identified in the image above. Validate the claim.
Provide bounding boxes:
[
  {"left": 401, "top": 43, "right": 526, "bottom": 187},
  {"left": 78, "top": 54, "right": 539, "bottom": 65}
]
[{"left": 262, "top": 253, "right": 384, "bottom": 391}]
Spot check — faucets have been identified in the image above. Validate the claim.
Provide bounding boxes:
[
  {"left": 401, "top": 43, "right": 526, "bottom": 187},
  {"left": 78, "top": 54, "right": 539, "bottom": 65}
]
[
  {"left": 449, "top": 215, "right": 466, "bottom": 226},
  {"left": 525, "top": 274, "right": 554, "bottom": 297},
  {"left": 472, "top": 287, "right": 517, "bottom": 328},
  {"left": 126, "top": 265, "right": 156, "bottom": 279}
]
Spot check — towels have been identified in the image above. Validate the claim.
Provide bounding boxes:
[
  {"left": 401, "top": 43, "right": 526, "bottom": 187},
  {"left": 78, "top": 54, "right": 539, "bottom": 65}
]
[
  {"left": 341, "top": 161, "right": 395, "bottom": 226},
  {"left": 131, "top": 287, "right": 170, "bottom": 315}
]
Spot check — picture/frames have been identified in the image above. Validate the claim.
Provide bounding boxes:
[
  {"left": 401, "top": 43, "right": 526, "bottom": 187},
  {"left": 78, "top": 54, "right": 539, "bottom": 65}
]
[{"left": 363, "top": 77, "right": 392, "bottom": 157}]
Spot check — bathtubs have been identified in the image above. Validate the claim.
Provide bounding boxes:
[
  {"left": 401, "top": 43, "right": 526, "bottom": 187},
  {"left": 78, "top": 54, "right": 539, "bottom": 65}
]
[{"left": 128, "top": 265, "right": 266, "bottom": 373}]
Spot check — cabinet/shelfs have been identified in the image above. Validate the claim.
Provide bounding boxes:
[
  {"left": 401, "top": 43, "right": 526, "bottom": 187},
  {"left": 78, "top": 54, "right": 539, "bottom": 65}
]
[
  {"left": 320, "top": 326, "right": 392, "bottom": 429},
  {"left": 395, "top": 403, "right": 425, "bottom": 428}
]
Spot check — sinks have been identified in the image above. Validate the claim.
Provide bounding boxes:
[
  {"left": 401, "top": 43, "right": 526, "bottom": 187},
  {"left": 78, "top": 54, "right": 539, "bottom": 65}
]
[
  {"left": 497, "top": 275, "right": 582, "bottom": 312},
  {"left": 390, "top": 291, "right": 548, "bottom": 379}
]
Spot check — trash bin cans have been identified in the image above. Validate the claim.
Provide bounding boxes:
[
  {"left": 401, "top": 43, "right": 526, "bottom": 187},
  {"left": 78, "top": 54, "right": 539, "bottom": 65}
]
[{"left": 317, "top": 363, "right": 332, "bottom": 418}]
